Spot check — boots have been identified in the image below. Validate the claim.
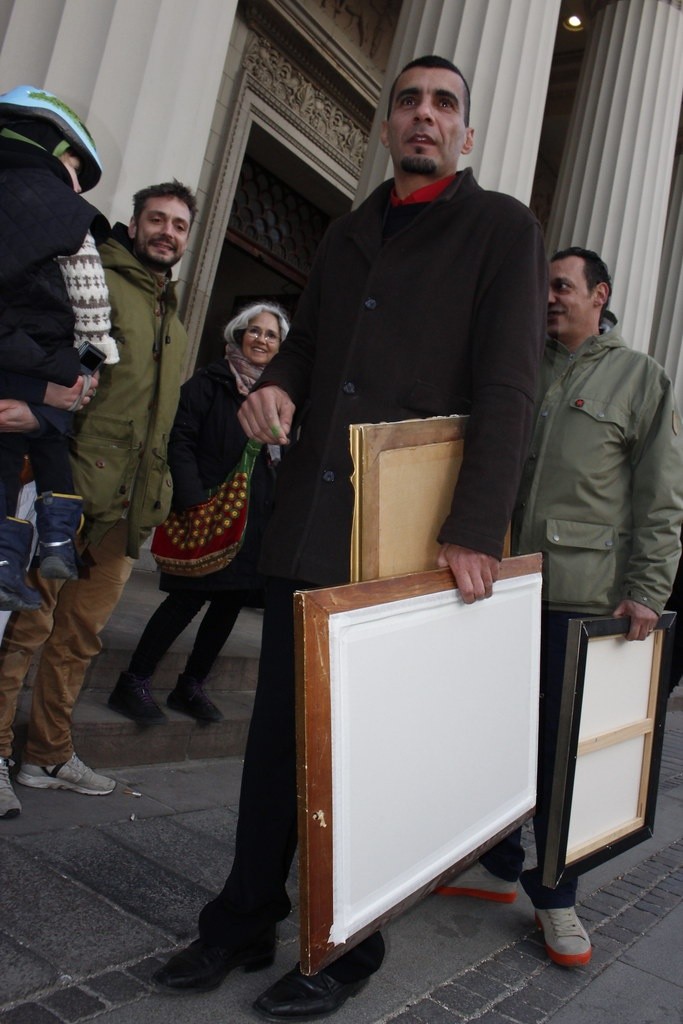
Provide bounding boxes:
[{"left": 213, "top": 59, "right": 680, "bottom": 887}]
[
  {"left": 0, "top": 515, "right": 41, "bottom": 610},
  {"left": 30, "top": 493, "right": 85, "bottom": 579}
]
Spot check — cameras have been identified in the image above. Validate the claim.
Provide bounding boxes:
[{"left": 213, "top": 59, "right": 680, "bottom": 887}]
[{"left": 76, "top": 341, "right": 107, "bottom": 378}]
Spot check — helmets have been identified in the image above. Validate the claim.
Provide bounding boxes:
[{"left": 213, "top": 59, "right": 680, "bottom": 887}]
[{"left": 0, "top": 86, "right": 103, "bottom": 191}]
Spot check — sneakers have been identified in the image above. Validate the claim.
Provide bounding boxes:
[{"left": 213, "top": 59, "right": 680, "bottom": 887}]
[
  {"left": 0, "top": 758, "right": 22, "bottom": 817},
  {"left": 106, "top": 672, "right": 167, "bottom": 724},
  {"left": 532, "top": 903, "right": 591, "bottom": 965},
  {"left": 167, "top": 674, "right": 224, "bottom": 724},
  {"left": 16, "top": 750, "right": 116, "bottom": 794},
  {"left": 431, "top": 865, "right": 518, "bottom": 903}
]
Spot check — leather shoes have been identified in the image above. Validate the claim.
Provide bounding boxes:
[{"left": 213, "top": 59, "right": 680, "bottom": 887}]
[
  {"left": 151, "top": 939, "right": 279, "bottom": 993},
  {"left": 252, "top": 964, "right": 370, "bottom": 1024}
]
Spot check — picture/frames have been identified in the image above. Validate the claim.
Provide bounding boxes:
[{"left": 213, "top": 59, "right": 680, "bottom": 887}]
[
  {"left": 350, "top": 416, "right": 466, "bottom": 582},
  {"left": 289, "top": 553, "right": 542, "bottom": 980},
  {"left": 541, "top": 610, "right": 676, "bottom": 887}
]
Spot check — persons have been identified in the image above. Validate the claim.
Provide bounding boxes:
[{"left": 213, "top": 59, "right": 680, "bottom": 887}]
[
  {"left": 427, "top": 245, "right": 682, "bottom": 967},
  {"left": 151, "top": 57, "right": 547, "bottom": 1024},
  {"left": 108, "top": 301, "right": 290, "bottom": 726},
  {"left": 0, "top": 84, "right": 121, "bottom": 654},
  {"left": 0, "top": 177, "right": 199, "bottom": 814}
]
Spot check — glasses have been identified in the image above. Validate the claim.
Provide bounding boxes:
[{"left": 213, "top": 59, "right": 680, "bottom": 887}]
[{"left": 243, "top": 326, "right": 282, "bottom": 343}]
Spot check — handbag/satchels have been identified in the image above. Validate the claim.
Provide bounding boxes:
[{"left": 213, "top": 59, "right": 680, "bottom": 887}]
[{"left": 149, "top": 467, "right": 253, "bottom": 573}]
